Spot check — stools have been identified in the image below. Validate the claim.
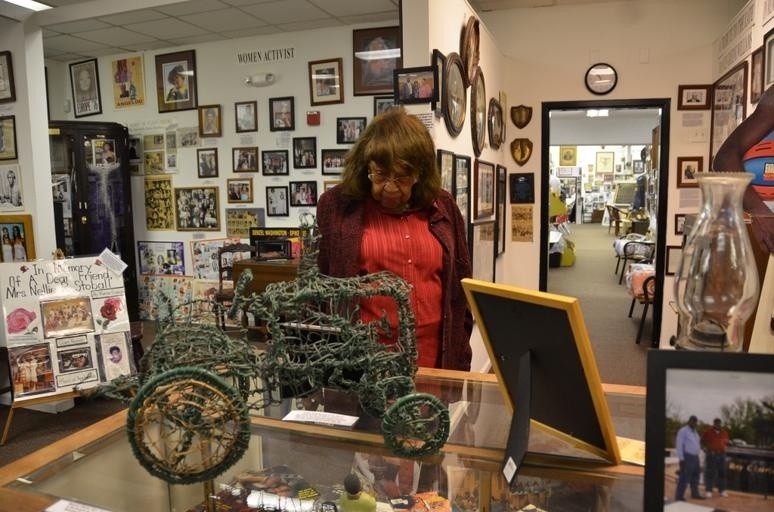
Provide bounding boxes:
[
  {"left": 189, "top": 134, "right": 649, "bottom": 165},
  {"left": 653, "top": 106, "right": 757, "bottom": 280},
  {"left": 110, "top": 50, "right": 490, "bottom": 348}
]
[{"left": 130, "top": 323, "right": 153, "bottom": 381}]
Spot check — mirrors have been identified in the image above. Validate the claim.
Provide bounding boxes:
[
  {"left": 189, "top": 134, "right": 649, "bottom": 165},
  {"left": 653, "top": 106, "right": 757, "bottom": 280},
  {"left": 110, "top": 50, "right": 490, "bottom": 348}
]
[{"left": 540, "top": 97, "right": 671, "bottom": 467}]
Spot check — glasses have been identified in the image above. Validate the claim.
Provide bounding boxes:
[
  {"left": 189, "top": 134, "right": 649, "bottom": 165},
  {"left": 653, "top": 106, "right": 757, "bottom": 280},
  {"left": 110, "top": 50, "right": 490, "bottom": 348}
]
[{"left": 367, "top": 165, "right": 418, "bottom": 186}]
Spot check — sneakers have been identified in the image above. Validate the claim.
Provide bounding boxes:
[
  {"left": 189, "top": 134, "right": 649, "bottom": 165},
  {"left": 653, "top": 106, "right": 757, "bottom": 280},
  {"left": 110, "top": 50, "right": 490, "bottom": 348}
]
[{"left": 677, "top": 491, "right": 728, "bottom": 501}]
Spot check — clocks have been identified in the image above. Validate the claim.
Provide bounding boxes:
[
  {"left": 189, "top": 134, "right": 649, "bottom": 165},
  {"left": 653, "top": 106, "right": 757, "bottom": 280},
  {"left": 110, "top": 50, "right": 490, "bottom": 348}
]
[{"left": 585, "top": 63, "right": 617, "bottom": 95}]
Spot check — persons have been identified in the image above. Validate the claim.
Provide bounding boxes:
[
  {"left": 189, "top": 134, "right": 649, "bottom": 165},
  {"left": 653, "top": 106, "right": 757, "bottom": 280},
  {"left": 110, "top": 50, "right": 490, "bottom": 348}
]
[
  {"left": 316, "top": 107, "right": 474, "bottom": 434},
  {"left": 78, "top": 39, "right": 433, "bottom": 274},
  {"left": 338, "top": 444, "right": 451, "bottom": 512},
  {"left": 684, "top": 165, "right": 696, "bottom": 179},
  {"left": 675, "top": 415, "right": 729, "bottom": 501},
  {"left": 1, "top": 169, "right": 27, "bottom": 263},
  {"left": 15, "top": 302, "right": 123, "bottom": 393},
  {"left": 687, "top": 93, "right": 699, "bottom": 103}
]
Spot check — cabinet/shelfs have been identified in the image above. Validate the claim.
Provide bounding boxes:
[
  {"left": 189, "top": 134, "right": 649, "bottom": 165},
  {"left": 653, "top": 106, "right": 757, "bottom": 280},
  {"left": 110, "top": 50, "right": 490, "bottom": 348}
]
[{"left": 0, "top": 350, "right": 647, "bottom": 512}]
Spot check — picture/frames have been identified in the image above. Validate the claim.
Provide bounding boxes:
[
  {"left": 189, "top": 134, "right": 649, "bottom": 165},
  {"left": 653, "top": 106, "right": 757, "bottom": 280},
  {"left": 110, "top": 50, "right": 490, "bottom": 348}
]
[
  {"left": 666, "top": 245, "right": 684, "bottom": 275},
  {"left": 644, "top": 349, "right": 774, "bottom": 512},
  {"left": 677, "top": 28, "right": 774, "bottom": 188},
  {"left": 432, "top": 16, "right": 509, "bottom": 285},
  {"left": 461, "top": 278, "right": 620, "bottom": 485},
  {"left": 559, "top": 125, "right": 659, "bottom": 213},
  {"left": 0, "top": 50, "right": 36, "bottom": 263},
  {"left": 676, "top": 214, "right": 700, "bottom": 234}
]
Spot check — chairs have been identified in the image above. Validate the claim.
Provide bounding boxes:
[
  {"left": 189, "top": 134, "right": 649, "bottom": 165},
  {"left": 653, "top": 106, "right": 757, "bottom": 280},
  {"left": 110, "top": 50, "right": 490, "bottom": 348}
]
[
  {"left": 629, "top": 258, "right": 655, "bottom": 344},
  {"left": 614, "top": 233, "right": 655, "bottom": 285},
  {"left": 606, "top": 205, "right": 628, "bottom": 236}
]
